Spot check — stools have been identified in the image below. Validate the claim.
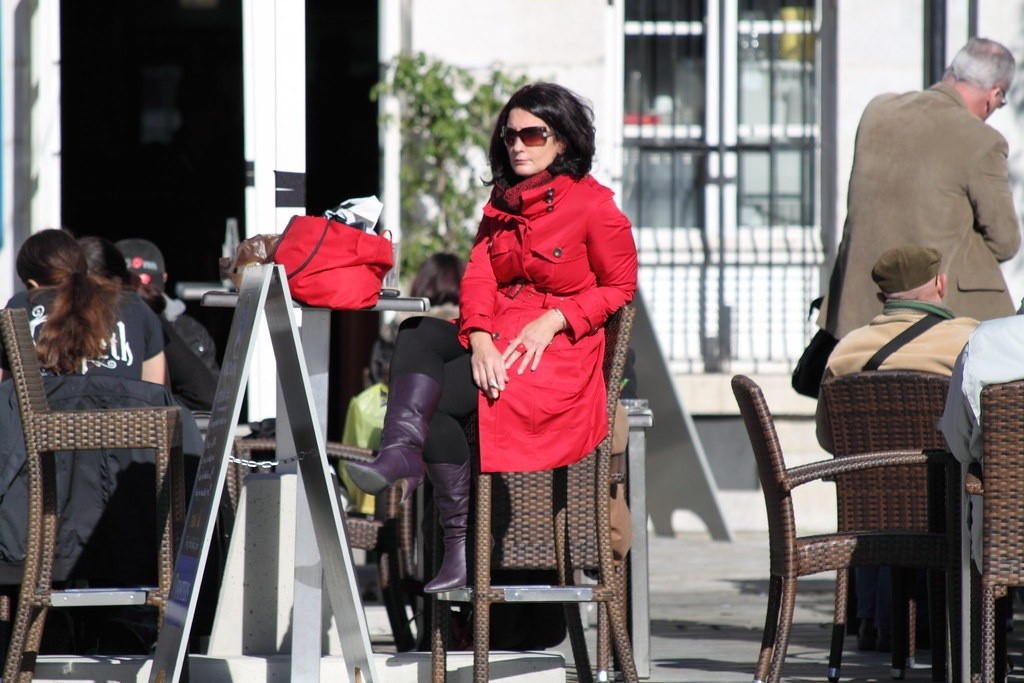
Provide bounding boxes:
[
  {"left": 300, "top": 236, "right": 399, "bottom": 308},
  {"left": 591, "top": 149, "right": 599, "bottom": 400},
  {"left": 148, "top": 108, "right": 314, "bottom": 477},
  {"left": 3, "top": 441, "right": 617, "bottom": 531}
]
[
  {"left": 429, "top": 300, "right": 637, "bottom": 683},
  {"left": 0, "top": 308, "right": 181, "bottom": 683}
]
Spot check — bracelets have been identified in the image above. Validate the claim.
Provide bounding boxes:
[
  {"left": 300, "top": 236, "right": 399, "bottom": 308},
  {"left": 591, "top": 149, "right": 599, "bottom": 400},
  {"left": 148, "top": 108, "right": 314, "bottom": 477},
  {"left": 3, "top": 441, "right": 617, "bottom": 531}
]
[{"left": 552, "top": 306, "right": 568, "bottom": 331}]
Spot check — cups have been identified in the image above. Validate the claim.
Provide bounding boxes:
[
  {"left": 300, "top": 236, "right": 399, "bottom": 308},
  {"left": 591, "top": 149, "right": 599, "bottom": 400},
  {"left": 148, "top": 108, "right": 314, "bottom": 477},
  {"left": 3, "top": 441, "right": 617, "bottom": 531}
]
[{"left": 383, "top": 243, "right": 399, "bottom": 289}]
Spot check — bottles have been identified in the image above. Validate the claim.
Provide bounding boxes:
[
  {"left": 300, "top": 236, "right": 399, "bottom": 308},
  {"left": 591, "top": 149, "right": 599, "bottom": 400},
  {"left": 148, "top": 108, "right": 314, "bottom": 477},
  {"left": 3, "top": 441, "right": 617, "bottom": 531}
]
[{"left": 220, "top": 219, "right": 240, "bottom": 290}]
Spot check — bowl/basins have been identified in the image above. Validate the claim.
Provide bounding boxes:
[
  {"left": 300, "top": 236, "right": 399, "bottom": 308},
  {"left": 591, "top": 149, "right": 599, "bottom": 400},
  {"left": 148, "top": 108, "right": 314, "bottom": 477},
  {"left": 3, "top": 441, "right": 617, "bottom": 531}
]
[{"left": 621, "top": 398, "right": 648, "bottom": 410}]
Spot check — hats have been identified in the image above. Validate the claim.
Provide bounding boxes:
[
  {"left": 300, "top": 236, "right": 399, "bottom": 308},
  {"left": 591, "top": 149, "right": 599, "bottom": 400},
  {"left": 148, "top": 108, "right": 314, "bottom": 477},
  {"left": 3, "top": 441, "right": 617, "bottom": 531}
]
[
  {"left": 113, "top": 238, "right": 168, "bottom": 296},
  {"left": 871, "top": 243, "right": 942, "bottom": 293}
]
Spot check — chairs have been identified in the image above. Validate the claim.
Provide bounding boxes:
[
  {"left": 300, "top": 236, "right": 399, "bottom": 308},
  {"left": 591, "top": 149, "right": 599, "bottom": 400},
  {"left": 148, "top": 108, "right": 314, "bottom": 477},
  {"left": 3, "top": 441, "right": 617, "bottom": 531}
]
[
  {"left": 730, "top": 371, "right": 945, "bottom": 683},
  {"left": 821, "top": 368, "right": 950, "bottom": 668},
  {"left": 961, "top": 376, "right": 1024, "bottom": 683}
]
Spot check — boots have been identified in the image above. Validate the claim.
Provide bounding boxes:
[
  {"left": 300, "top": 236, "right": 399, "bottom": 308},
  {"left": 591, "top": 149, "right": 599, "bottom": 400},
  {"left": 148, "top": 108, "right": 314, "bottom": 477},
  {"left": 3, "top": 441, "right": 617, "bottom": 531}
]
[
  {"left": 423, "top": 456, "right": 496, "bottom": 592},
  {"left": 344, "top": 372, "right": 444, "bottom": 507}
]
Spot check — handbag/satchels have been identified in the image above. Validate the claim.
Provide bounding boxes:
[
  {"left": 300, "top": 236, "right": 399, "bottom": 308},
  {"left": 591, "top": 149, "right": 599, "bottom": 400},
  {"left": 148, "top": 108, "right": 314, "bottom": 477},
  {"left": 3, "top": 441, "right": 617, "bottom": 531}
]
[
  {"left": 231, "top": 234, "right": 289, "bottom": 294},
  {"left": 265, "top": 214, "right": 394, "bottom": 311},
  {"left": 791, "top": 296, "right": 843, "bottom": 398},
  {"left": 336, "top": 382, "right": 391, "bottom": 515}
]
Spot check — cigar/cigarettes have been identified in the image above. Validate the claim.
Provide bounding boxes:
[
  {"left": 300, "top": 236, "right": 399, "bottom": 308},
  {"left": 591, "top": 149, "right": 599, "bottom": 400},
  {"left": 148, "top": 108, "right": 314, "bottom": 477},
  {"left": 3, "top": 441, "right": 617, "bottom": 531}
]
[{"left": 489, "top": 378, "right": 501, "bottom": 391}]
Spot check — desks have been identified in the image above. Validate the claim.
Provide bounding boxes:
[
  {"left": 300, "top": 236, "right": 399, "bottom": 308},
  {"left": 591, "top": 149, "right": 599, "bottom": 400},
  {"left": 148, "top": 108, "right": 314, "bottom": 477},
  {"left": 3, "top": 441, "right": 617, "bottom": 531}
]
[{"left": 182, "top": 289, "right": 431, "bottom": 683}]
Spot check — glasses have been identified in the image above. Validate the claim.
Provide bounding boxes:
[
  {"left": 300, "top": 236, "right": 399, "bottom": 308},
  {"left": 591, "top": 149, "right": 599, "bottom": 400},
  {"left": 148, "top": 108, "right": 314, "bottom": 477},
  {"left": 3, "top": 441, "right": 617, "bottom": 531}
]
[
  {"left": 999, "top": 88, "right": 1008, "bottom": 107},
  {"left": 500, "top": 125, "right": 559, "bottom": 146}
]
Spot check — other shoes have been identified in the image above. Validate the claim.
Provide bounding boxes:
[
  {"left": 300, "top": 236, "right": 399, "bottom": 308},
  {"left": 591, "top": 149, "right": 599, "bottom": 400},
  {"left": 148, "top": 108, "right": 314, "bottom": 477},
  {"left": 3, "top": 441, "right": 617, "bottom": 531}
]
[{"left": 858, "top": 619, "right": 894, "bottom": 654}]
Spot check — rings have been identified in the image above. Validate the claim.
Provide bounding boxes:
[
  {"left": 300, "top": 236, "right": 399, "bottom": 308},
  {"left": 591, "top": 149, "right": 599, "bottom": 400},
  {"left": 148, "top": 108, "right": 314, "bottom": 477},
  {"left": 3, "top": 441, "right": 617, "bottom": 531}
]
[{"left": 515, "top": 343, "right": 527, "bottom": 353}]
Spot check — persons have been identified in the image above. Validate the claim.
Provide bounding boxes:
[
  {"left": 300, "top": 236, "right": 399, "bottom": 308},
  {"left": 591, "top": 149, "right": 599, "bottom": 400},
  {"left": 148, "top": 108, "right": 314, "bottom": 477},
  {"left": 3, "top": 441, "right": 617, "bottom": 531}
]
[
  {"left": 816, "top": 39, "right": 1021, "bottom": 344},
  {"left": 816, "top": 243, "right": 1024, "bottom": 676},
  {"left": 0, "top": 229, "right": 219, "bottom": 655},
  {"left": 370, "top": 251, "right": 468, "bottom": 395},
  {"left": 343, "top": 85, "right": 638, "bottom": 593}
]
